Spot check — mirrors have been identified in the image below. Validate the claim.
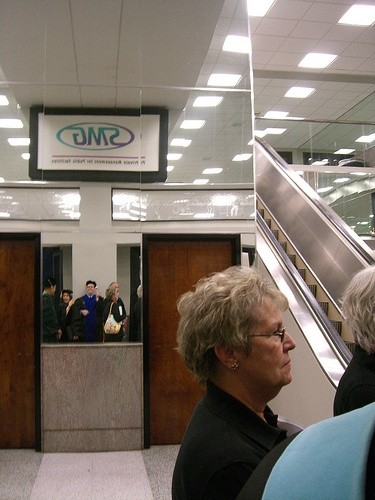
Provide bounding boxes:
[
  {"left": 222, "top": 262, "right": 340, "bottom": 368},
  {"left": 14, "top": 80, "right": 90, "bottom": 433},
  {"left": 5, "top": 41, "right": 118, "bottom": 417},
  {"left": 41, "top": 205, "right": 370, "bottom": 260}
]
[{"left": 40, "top": 231, "right": 143, "bottom": 347}]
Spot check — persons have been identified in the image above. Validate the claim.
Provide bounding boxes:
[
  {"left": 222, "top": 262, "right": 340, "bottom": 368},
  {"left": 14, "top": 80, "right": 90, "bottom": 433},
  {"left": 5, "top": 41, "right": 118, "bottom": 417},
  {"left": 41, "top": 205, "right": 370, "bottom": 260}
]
[
  {"left": 129, "top": 285, "right": 141, "bottom": 341},
  {"left": 236, "top": 401, "right": 375, "bottom": 500},
  {"left": 42, "top": 277, "right": 126, "bottom": 343},
  {"left": 170, "top": 266, "right": 295, "bottom": 500},
  {"left": 333, "top": 265, "right": 375, "bottom": 416}
]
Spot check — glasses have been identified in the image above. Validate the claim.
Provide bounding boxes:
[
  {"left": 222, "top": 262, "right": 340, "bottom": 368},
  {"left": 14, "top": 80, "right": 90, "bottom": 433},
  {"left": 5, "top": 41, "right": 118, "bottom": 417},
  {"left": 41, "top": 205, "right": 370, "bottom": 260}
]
[{"left": 249, "top": 328, "right": 285, "bottom": 343}]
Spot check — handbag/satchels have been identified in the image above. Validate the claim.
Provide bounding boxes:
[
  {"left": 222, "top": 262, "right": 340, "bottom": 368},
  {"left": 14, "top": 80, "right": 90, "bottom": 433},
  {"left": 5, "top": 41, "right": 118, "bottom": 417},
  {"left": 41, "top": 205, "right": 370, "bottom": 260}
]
[{"left": 104, "top": 303, "right": 122, "bottom": 334}]
